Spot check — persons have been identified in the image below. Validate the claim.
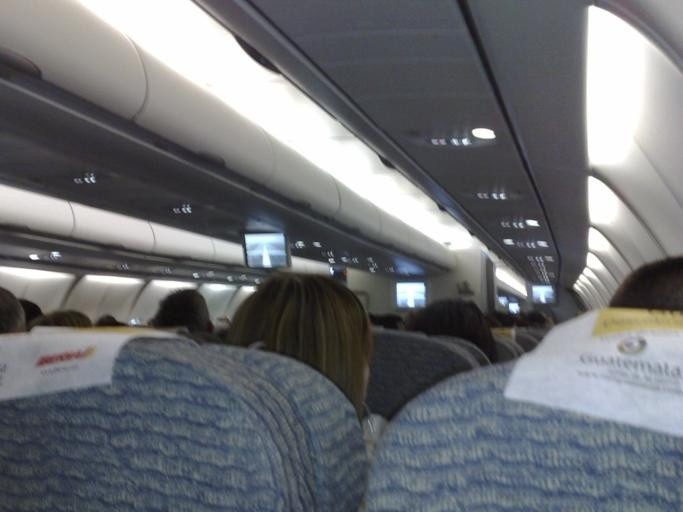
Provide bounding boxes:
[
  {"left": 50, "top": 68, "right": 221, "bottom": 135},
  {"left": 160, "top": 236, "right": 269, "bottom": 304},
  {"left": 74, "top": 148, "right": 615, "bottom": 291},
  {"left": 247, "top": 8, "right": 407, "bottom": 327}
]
[{"left": 1, "top": 257, "right": 683, "bottom": 457}]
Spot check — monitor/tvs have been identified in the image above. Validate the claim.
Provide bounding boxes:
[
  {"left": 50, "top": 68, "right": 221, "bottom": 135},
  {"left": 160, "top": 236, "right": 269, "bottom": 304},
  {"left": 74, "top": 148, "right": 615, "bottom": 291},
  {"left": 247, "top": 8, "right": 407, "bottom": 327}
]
[
  {"left": 497, "top": 296, "right": 520, "bottom": 314},
  {"left": 241, "top": 231, "right": 292, "bottom": 269},
  {"left": 395, "top": 281, "right": 428, "bottom": 311},
  {"left": 531, "top": 283, "right": 558, "bottom": 306}
]
